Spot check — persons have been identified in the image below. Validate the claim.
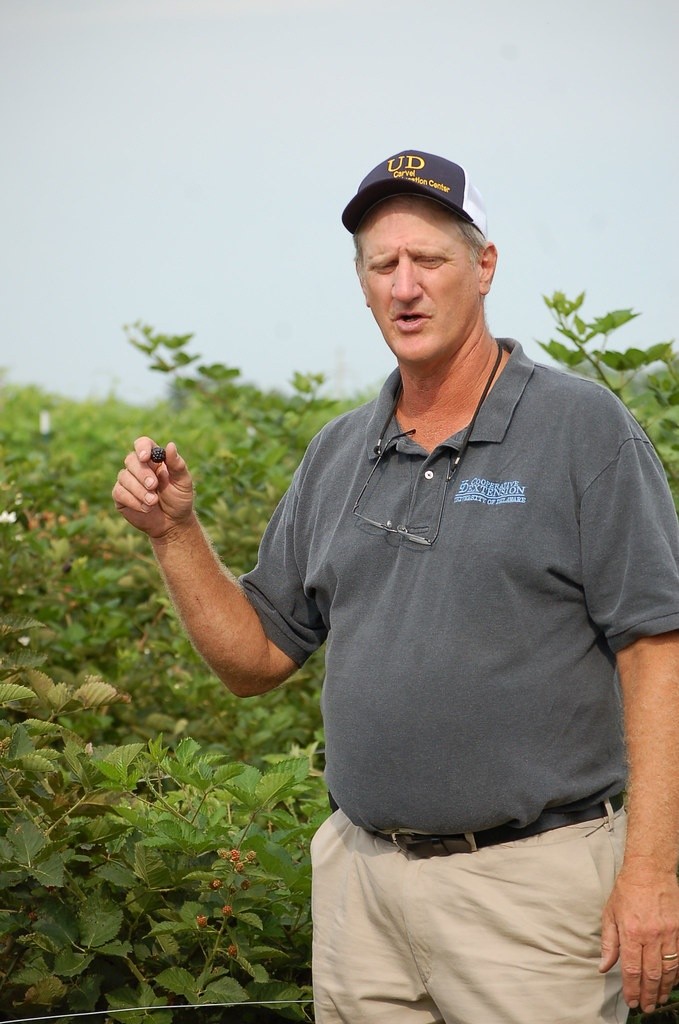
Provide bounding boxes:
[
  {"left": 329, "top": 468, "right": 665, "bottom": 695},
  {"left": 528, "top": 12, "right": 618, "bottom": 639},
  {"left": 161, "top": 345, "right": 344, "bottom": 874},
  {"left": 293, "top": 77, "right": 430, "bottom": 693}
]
[{"left": 112, "top": 149, "right": 679, "bottom": 1024}]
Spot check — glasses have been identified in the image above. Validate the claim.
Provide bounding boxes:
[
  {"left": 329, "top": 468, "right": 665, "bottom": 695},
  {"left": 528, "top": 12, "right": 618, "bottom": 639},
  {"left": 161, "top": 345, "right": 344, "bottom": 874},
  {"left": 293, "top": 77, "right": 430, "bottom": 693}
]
[{"left": 351, "top": 429, "right": 455, "bottom": 546}]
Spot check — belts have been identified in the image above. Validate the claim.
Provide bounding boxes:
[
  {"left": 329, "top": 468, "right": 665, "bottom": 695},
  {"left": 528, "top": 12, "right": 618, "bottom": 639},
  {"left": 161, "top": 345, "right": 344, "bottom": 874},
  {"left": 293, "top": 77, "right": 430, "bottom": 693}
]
[{"left": 363, "top": 791, "right": 623, "bottom": 859}]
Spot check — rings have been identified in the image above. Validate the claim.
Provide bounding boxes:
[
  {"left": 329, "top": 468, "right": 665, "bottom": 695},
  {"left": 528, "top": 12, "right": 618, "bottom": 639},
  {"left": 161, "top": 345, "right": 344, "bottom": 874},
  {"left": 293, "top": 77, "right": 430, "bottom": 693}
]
[{"left": 661, "top": 951, "right": 679, "bottom": 961}]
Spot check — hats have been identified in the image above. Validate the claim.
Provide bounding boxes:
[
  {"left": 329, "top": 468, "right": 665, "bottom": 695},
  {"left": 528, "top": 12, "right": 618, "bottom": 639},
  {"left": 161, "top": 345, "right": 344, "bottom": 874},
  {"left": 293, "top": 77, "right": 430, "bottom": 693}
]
[{"left": 342, "top": 150, "right": 488, "bottom": 241}]
